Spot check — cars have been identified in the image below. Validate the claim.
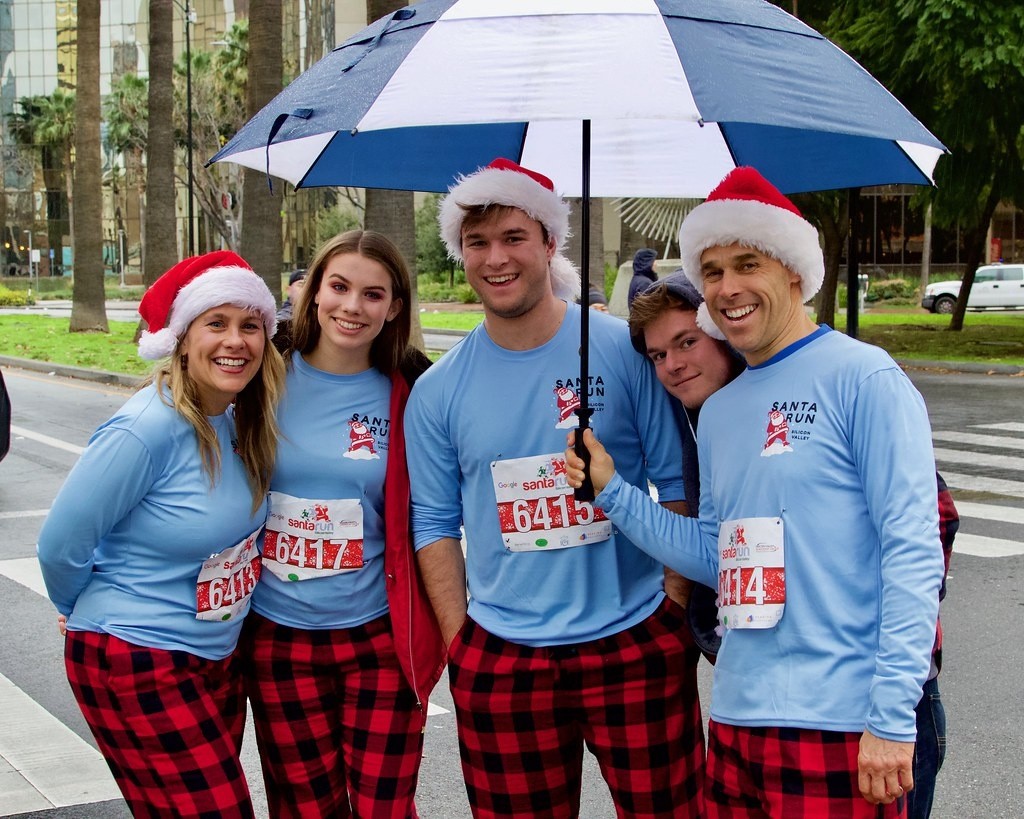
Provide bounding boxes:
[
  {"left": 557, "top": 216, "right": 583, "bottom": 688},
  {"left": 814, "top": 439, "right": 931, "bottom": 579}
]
[{"left": 921, "top": 261, "right": 1024, "bottom": 315}]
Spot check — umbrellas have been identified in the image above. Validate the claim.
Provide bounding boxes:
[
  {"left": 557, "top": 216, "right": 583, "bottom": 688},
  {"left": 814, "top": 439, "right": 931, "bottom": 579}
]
[{"left": 204, "top": 0, "right": 953, "bottom": 503}]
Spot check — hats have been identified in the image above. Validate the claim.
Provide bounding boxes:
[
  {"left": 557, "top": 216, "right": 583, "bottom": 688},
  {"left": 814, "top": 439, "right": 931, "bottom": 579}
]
[
  {"left": 437, "top": 157, "right": 581, "bottom": 302},
  {"left": 679, "top": 165, "right": 826, "bottom": 341},
  {"left": 138, "top": 249, "right": 280, "bottom": 361},
  {"left": 289, "top": 269, "right": 309, "bottom": 286},
  {"left": 576, "top": 285, "right": 607, "bottom": 307}
]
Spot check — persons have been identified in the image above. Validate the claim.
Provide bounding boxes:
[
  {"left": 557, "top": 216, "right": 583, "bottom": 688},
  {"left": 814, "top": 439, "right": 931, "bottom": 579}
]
[{"left": 39, "top": 156, "right": 959, "bottom": 819}]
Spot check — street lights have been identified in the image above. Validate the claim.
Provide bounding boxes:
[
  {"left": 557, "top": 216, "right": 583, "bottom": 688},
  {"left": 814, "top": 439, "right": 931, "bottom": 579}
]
[
  {"left": 226, "top": 218, "right": 237, "bottom": 253},
  {"left": 117, "top": 228, "right": 125, "bottom": 287},
  {"left": 24, "top": 230, "right": 32, "bottom": 300}
]
